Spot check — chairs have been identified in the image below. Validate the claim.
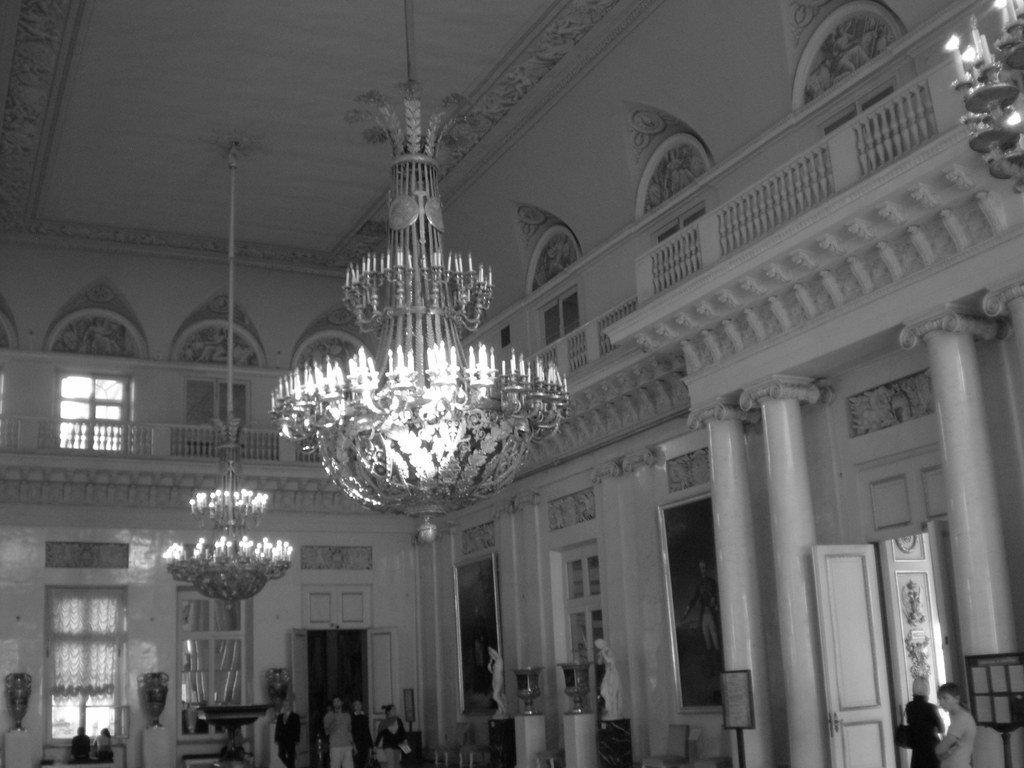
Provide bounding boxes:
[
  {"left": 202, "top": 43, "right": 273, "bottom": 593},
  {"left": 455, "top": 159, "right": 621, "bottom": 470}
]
[
  {"left": 434, "top": 722, "right": 469, "bottom": 768},
  {"left": 458, "top": 723, "right": 490, "bottom": 768},
  {"left": 641, "top": 723, "right": 688, "bottom": 768},
  {"left": 694, "top": 725, "right": 732, "bottom": 768},
  {"left": 535, "top": 725, "right": 564, "bottom": 768}
]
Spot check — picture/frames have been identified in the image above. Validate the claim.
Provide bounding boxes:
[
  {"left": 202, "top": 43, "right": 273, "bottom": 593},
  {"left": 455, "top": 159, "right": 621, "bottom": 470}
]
[
  {"left": 656, "top": 489, "right": 724, "bottom": 715},
  {"left": 452, "top": 553, "right": 503, "bottom": 716}
]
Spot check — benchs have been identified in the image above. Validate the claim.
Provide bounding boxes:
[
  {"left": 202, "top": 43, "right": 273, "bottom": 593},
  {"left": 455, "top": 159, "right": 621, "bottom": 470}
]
[
  {"left": 40, "top": 760, "right": 116, "bottom": 768},
  {"left": 181, "top": 754, "right": 254, "bottom": 768}
]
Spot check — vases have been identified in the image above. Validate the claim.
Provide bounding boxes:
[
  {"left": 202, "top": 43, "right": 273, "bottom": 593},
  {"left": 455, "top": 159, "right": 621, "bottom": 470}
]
[{"left": 185, "top": 702, "right": 198, "bottom": 734}]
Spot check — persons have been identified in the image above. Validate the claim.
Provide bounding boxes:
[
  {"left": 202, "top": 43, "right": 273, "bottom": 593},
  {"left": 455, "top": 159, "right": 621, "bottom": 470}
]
[
  {"left": 487, "top": 648, "right": 507, "bottom": 718},
  {"left": 373, "top": 704, "right": 408, "bottom": 768},
  {"left": 323, "top": 697, "right": 373, "bottom": 768},
  {"left": 274, "top": 700, "right": 301, "bottom": 768},
  {"left": 935, "top": 683, "right": 977, "bottom": 768},
  {"left": 91, "top": 728, "right": 115, "bottom": 764},
  {"left": 905, "top": 677, "right": 946, "bottom": 768},
  {"left": 68, "top": 727, "right": 91, "bottom": 764},
  {"left": 594, "top": 638, "right": 623, "bottom": 719}
]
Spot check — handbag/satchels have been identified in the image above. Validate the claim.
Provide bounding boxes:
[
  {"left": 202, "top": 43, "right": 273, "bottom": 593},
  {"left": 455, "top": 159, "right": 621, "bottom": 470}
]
[
  {"left": 895, "top": 710, "right": 913, "bottom": 749},
  {"left": 369, "top": 749, "right": 381, "bottom": 768}
]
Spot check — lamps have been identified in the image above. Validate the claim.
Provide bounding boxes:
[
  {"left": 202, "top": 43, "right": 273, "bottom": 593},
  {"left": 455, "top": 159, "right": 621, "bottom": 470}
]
[
  {"left": 944, "top": 0, "right": 1024, "bottom": 192},
  {"left": 272, "top": 0, "right": 572, "bottom": 538},
  {"left": 163, "top": 135, "right": 294, "bottom": 609}
]
